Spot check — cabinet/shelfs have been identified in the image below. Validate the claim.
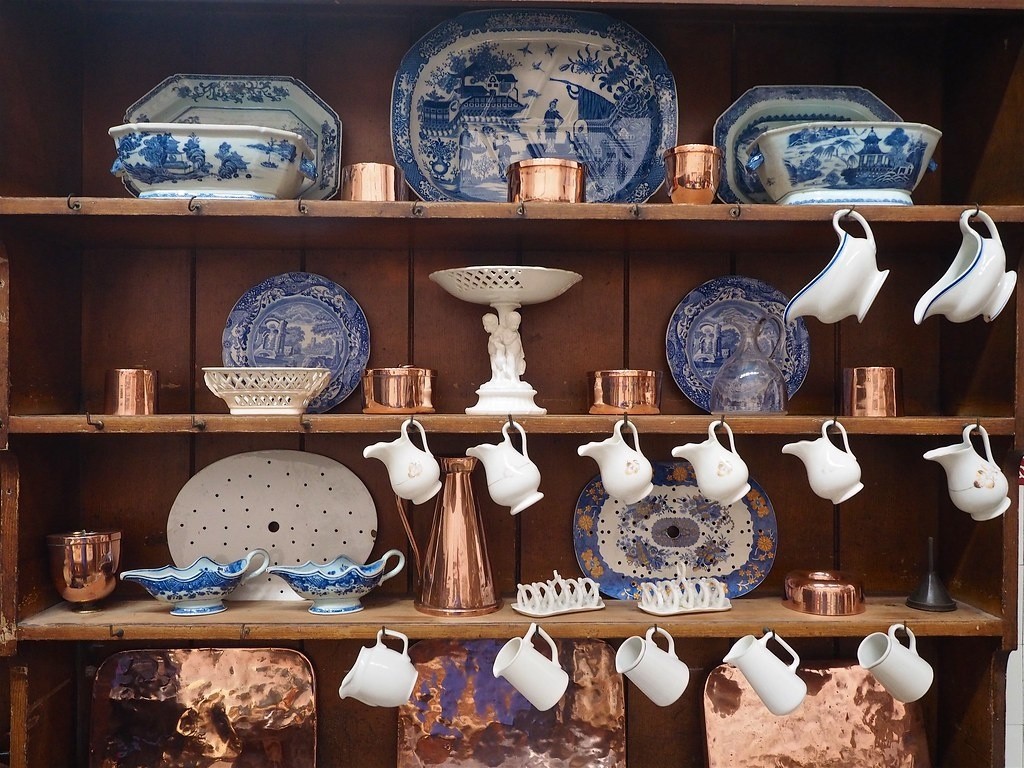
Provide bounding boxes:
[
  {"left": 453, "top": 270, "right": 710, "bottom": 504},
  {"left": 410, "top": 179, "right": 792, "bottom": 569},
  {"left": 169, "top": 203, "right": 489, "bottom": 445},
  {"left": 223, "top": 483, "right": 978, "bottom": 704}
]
[{"left": 0, "top": 0, "right": 1023, "bottom": 767}]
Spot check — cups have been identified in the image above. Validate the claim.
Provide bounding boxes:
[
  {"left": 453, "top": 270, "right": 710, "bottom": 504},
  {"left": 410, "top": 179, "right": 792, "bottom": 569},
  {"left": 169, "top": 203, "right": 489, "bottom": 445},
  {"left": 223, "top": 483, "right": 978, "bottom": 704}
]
[
  {"left": 616, "top": 627, "right": 690, "bottom": 708},
  {"left": 106, "top": 366, "right": 160, "bottom": 416},
  {"left": 662, "top": 144, "right": 726, "bottom": 204},
  {"left": 341, "top": 163, "right": 396, "bottom": 203},
  {"left": 840, "top": 368, "right": 896, "bottom": 418}
]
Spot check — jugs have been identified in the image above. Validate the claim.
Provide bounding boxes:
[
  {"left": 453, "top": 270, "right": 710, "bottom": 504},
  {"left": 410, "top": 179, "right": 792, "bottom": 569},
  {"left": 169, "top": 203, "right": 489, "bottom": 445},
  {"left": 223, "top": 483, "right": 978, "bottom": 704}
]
[
  {"left": 923, "top": 423, "right": 1012, "bottom": 521},
  {"left": 577, "top": 420, "right": 654, "bottom": 504},
  {"left": 671, "top": 421, "right": 752, "bottom": 507},
  {"left": 339, "top": 632, "right": 418, "bottom": 708},
  {"left": 782, "top": 420, "right": 863, "bottom": 505},
  {"left": 466, "top": 421, "right": 544, "bottom": 516},
  {"left": 722, "top": 635, "right": 808, "bottom": 717},
  {"left": 395, "top": 454, "right": 505, "bottom": 617},
  {"left": 493, "top": 623, "right": 568, "bottom": 712},
  {"left": 364, "top": 419, "right": 440, "bottom": 505},
  {"left": 783, "top": 209, "right": 890, "bottom": 327},
  {"left": 858, "top": 624, "right": 934, "bottom": 702},
  {"left": 913, "top": 210, "right": 1017, "bottom": 326}
]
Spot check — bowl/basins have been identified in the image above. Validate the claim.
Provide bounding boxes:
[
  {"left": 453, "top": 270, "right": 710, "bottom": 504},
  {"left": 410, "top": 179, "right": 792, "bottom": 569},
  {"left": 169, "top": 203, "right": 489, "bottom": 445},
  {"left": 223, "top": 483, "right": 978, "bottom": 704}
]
[
  {"left": 585, "top": 369, "right": 663, "bottom": 415},
  {"left": 504, "top": 158, "right": 584, "bottom": 203},
  {"left": 747, "top": 121, "right": 943, "bottom": 205},
  {"left": 266, "top": 551, "right": 405, "bottom": 616},
  {"left": 120, "top": 549, "right": 269, "bottom": 616},
  {"left": 361, "top": 364, "right": 436, "bottom": 413},
  {"left": 110, "top": 124, "right": 316, "bottom": 201}
]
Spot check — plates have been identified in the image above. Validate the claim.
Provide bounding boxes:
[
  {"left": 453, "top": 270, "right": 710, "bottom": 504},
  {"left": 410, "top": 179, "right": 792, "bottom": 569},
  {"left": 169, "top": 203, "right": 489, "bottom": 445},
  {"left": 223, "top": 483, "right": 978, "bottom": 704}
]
[
  {"left": 712, "top": 86, "right": 903, "bottom": 209},
  {"left": 391, "top": 11, "right": 678, "bottom": 204},
  {"left": 222, "top": 271, "right": 370, "bottom": 413},
  {"left": 665, "top": 276, "right": 811, "bottom": 416},
  {"left": 121, "top": 74, "right": 342, "bottom": 201}
]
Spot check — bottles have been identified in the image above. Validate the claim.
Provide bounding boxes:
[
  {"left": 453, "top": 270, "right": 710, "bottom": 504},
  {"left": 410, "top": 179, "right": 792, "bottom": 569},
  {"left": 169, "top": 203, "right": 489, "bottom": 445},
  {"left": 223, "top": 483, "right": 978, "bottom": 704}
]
[{"left": 710, "top": 321, "right": 793, "bottom": 415}]
